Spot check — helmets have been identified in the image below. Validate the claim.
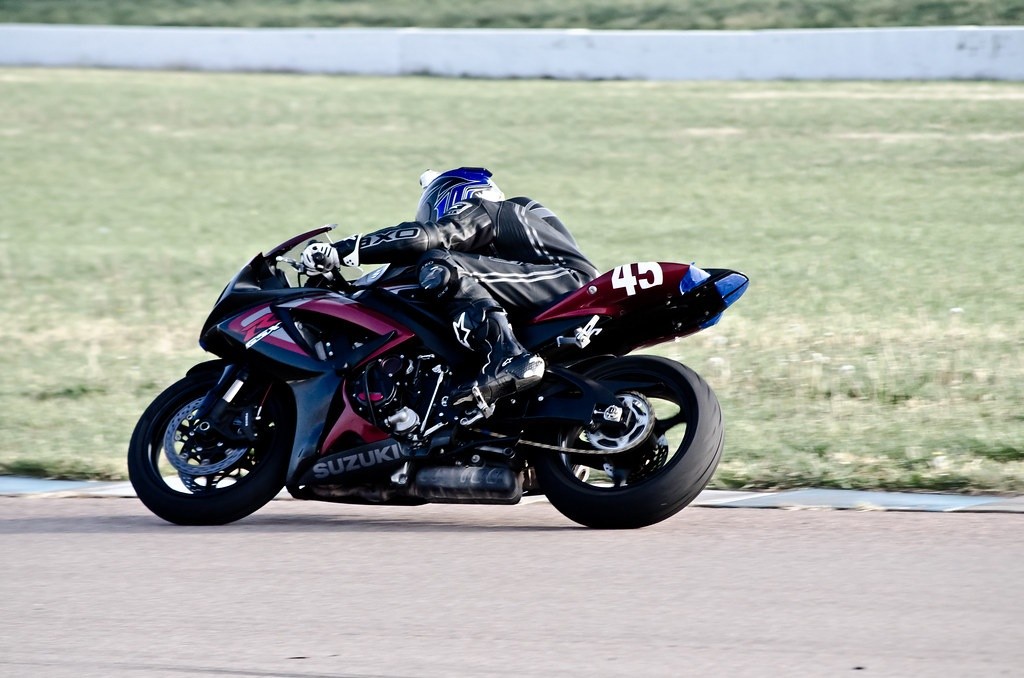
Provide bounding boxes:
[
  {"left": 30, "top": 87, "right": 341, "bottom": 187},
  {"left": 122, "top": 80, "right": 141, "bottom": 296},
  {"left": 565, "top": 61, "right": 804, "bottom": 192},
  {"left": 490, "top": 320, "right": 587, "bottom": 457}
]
[{"left": 415, "top": 167, "right": 505, "bottom": 224}]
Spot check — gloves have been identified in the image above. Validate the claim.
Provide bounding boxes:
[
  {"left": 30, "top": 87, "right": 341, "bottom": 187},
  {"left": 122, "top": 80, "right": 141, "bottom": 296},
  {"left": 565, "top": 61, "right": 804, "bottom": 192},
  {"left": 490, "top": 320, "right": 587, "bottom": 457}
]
[{"left": 299, "top": 232, "right": 362, "bottom": 275}]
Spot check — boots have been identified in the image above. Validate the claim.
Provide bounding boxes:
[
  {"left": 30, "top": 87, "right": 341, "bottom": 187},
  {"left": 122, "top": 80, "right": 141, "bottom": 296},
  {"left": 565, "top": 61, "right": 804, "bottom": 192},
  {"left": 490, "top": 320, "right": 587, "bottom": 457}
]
[{"left": 446, "top": 297, "right": 546, "bottom": 421}]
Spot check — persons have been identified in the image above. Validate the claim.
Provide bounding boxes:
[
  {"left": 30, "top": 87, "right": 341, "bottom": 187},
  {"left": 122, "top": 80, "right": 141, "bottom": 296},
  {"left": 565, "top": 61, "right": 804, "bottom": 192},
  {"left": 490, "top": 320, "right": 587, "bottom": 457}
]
[{"left": 303, "top": 167, "right": 598, "bottom": 409}]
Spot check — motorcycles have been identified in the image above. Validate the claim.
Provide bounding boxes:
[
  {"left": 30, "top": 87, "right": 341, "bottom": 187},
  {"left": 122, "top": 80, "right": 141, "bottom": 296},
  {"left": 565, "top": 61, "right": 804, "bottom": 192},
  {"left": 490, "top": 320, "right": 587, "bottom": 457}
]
[{"left": 127, "top": 222, "right": 750, "bottom": 530}]
